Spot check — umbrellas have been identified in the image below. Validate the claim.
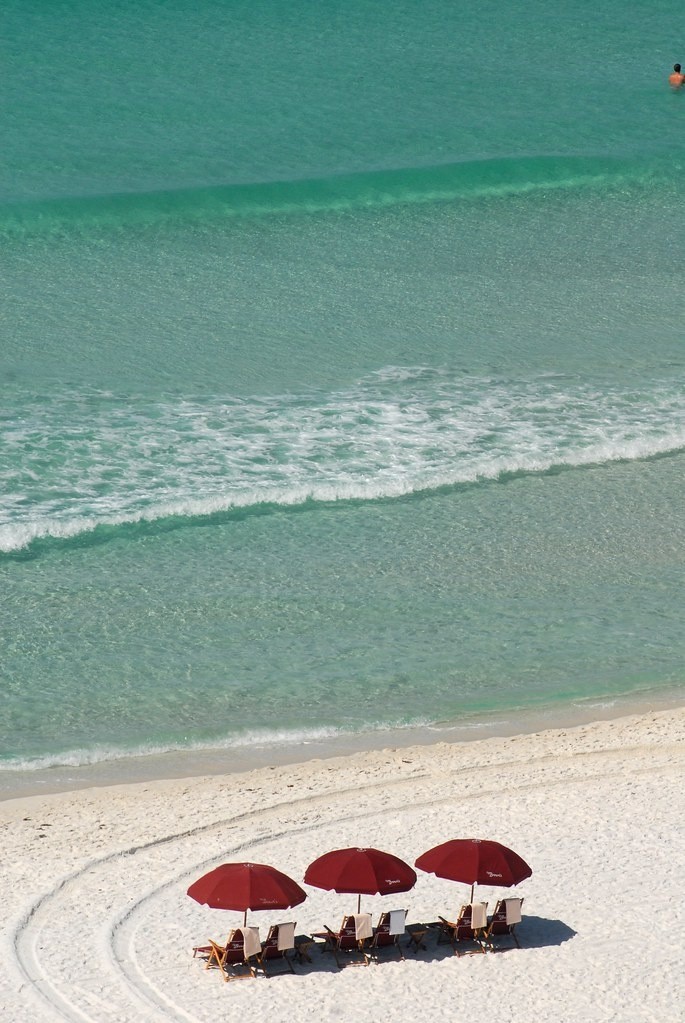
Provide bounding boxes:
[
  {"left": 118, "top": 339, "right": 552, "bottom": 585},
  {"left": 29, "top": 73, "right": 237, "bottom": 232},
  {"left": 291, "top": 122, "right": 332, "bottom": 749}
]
[
  {"left": 188, "top": 863, "right": 306, "bottom": 929},
  {"left": 304, "top": 847, "right": 416, "bottom": 915},
  {"left": 413, "top": 838, "right": 532, "bottom": 904}
]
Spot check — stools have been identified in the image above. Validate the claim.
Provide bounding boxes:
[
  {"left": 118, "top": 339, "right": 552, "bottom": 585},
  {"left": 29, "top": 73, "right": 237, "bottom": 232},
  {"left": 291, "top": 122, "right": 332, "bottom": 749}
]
[
  {"left": 403, "top": 923, "right": 430, "bottom": 954},
  {"left": 293, "top": 934, "right": 315, "bottom": 963}
]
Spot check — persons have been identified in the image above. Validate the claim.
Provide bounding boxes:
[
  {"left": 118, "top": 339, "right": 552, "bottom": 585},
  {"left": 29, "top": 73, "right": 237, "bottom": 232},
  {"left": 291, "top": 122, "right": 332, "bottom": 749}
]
[{"left": 669, "top": 63, "right": 685, "bottom": 89}]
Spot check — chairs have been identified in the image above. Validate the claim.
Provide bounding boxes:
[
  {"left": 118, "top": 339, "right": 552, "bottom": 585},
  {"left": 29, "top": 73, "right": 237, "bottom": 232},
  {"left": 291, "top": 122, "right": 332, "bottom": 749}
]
[
  {"left": 251, "top": 922, "right": 297, "bottom": 978},
  {"left": 361, "top": 909, "right": 409, "bottom": 963},
  {"left": 480, "top": 898, "right": 525, "bottom": 953},
  {"left": 435, "top": 901, "right": 489, "bottom": 958},
  {"left": 311, "top": 912, "right": 372, "bottom": 969},
  {"left": 192, "top": 926, "right": 259, "bottom": 982}
]
